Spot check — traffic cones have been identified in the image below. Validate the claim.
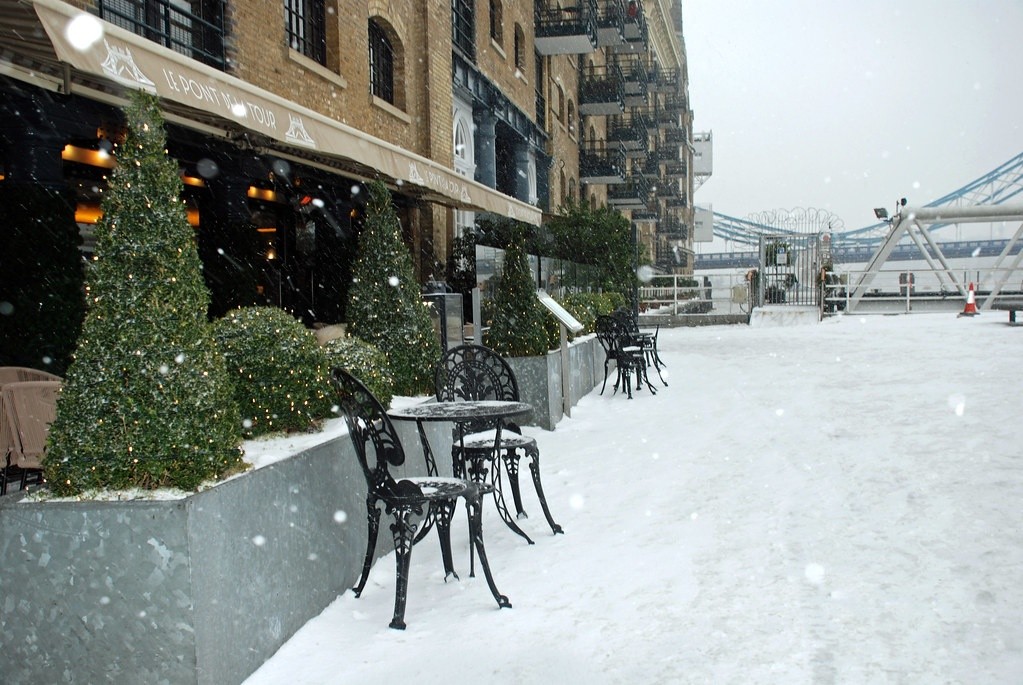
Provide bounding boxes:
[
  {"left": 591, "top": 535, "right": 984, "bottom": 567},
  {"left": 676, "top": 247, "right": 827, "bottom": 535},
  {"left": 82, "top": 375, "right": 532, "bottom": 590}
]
[{"left": 960, "top": 282, "right": 980, "bottom": 317}]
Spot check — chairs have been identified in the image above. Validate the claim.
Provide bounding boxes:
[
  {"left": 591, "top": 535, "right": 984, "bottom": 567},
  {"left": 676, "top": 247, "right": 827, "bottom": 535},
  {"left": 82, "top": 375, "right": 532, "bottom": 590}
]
[
  {"left": 331, "top": 368, "right": 512, "bottom": 629},
  {"left": 0, "top": 366, "right": 64, "bottom": 495},
  {"left": 433, "top": 344, "right": 564, "bottom": 535},
  {"left": 595, "top": 306, "right": 668, "bottom": 399},
  {"left": 3, "top": 381, "right": 63, "bottom": 491}
]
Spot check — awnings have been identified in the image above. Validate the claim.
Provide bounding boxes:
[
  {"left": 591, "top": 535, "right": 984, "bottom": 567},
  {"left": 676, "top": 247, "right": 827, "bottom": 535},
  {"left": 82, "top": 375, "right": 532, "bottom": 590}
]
[{"left": 0, "top": 0, "right": 545, "bottom": 228}]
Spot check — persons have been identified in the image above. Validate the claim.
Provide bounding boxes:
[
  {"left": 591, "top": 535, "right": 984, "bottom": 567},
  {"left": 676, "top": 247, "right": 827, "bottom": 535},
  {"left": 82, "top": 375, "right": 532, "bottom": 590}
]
[{"left": 704, "top": 276, "right": 716, "bottom": 309}]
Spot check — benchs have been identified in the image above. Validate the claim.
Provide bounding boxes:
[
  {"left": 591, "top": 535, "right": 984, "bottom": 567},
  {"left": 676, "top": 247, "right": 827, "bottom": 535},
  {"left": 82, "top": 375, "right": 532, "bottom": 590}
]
[{"left": 990, "top": 300, "right": 1023, "bottom": 325}]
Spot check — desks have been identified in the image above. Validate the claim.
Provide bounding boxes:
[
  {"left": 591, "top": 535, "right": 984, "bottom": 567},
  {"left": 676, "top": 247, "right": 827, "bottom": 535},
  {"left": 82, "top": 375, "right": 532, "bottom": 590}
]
[
  {"left": 385, "top": 400, "right": 535, "bottom": 578},
  {"left": 595, "top": 332, "right": 669, "bottom": 399}
]
